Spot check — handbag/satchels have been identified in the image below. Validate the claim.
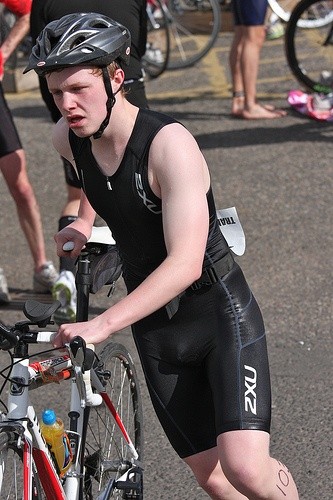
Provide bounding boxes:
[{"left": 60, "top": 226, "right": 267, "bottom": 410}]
[{"left": 265, "top": 19, "right": 285, "bottom": 40}]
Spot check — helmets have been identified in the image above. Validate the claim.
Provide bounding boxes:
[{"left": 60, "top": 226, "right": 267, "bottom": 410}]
[{"left": 22, "top": 11, "right": 133, "bottom": 76}]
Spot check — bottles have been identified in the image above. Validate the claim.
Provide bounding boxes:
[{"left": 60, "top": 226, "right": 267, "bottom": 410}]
[{"left": 40, "top": 409, "right": 73, "bottom": 478}]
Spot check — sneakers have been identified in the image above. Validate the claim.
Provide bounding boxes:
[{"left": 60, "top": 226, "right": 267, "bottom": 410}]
[
  {"left": 0, "top": 268, "right": 12, "bottom": 304},
  {"left": 51, "top": 271, "right": 79, "bottom": 328},
  {"left": 34, "top": 260, "right": 59, "bottom": 295}
]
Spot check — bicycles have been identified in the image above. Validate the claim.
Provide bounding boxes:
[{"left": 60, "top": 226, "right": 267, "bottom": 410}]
[
  {"left": 142, "top": 0, "right": 223, "bottom": 70},
  {"left": 267, "top": 1, "right": 332, "bottom": 29},
  {"left": 0, "top": 225, "right": 147, "bottom": 500},
  {"left": 144, "top": 0, "right": 172, "bottom": 81},
  {"left": 284, "top": 2, "right": 332, "bottom": 97}
]
